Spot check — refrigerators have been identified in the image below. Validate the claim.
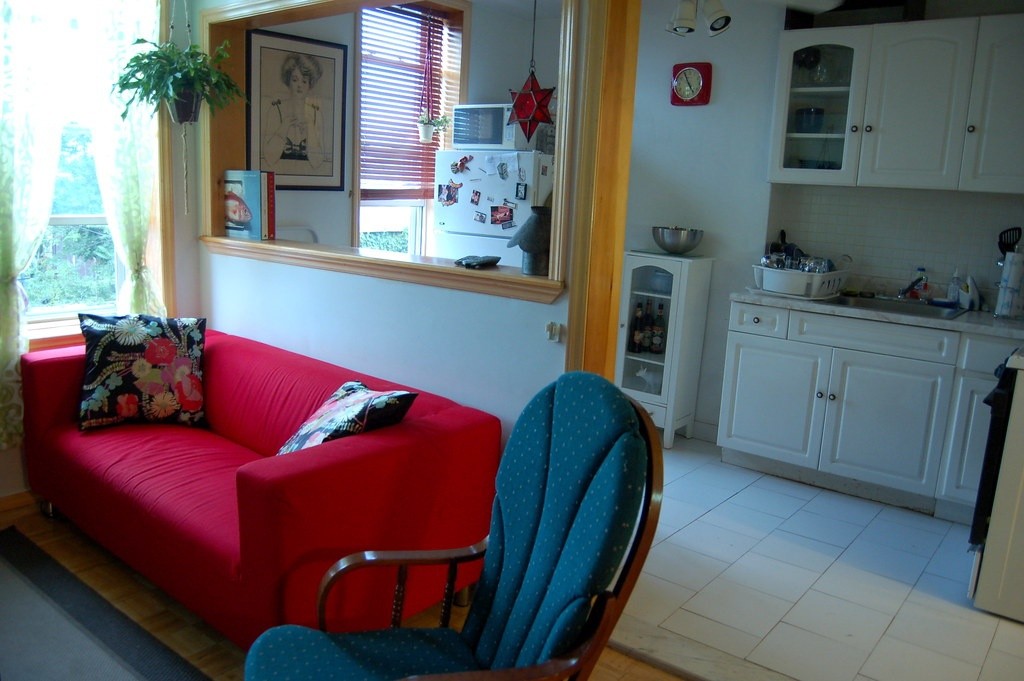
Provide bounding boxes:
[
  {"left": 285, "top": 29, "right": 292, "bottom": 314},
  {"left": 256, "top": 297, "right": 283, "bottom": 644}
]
[{"left": 430, "top": 150, "right": 554, "bottom": 267}]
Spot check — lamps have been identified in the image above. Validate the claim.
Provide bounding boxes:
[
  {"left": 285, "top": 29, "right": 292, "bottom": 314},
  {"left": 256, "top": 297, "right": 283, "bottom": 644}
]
[
  {"left": 664, "top": 0, "right": 697, "bottom": 38},
  {"left": 697, "top": 0, "right": 734, "bottom": 36}
]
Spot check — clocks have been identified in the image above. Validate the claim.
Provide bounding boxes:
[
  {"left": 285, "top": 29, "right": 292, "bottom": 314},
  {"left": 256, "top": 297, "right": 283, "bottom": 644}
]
[{"left": 670, "top": 63, "right": 712, "bottom": 106}]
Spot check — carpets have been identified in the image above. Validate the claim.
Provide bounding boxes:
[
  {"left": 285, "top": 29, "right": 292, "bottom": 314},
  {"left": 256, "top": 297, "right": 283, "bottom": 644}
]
[{"left": 0, "top": 524, "right": 212, "bottom": 681}]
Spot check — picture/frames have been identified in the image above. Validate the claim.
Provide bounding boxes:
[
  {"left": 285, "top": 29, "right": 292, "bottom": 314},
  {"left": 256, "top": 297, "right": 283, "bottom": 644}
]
[{"left": 247, "top": 27, "right": 348, "bottom": 190}]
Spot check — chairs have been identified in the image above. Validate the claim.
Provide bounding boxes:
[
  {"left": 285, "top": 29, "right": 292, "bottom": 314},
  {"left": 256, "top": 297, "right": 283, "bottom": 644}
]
[{"left": 245, "top": 371, "right": 665, "bottom": 681}]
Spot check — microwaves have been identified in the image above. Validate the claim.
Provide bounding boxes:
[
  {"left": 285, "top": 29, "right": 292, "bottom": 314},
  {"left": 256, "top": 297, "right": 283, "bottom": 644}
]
[{"left": 453, "top": 104, "right": 537, "bottom": 150}]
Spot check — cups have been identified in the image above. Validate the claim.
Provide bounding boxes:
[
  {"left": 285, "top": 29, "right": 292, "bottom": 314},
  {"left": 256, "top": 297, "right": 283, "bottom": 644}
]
[{"left": 795, "top": 108, "right": 824, "bottom": 132}]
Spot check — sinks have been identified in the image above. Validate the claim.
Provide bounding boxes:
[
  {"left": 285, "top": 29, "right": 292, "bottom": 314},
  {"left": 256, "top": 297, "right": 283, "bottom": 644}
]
[
  {"left": 871, "top": 300, "right": 969, "bottom": 320},
  {"left": 815, "top": 294, "right": 881, "bottom": 310}
]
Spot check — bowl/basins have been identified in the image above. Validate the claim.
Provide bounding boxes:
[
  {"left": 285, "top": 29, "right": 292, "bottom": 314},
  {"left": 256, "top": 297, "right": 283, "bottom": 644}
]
[{"left": 652, "top": 226, "right": 704, "bottom": 255}]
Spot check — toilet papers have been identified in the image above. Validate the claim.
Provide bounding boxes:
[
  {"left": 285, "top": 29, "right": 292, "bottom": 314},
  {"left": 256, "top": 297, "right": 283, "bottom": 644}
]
[{"left": 995, "top": 252, "right": 1024, "bottom": 316}]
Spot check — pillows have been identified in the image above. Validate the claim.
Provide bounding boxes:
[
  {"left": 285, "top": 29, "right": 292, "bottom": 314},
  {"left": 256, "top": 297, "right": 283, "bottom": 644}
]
[
  {"left": 79, "top": 313, "right": 208, "bottom": 430},
  {"left": 276, "top": 380, "right": 418, "bottom": 457}
]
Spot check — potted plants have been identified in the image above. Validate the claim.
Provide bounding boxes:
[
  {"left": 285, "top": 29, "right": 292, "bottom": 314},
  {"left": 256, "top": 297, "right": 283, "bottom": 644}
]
[
  {"left": 111, "top": 37, "right": 247, "bottom": 124},
  {"left": 416, "top": 111, "right": 448, "bottom": 142}
]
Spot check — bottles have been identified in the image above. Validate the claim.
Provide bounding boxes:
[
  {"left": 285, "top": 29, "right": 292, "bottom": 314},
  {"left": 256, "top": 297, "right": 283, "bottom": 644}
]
[
  {"left": 629, "top": 299, "right": 667, "bottom": 354},
  {"left": 914, "top": 267, "right": 929, "bottom": 298}
]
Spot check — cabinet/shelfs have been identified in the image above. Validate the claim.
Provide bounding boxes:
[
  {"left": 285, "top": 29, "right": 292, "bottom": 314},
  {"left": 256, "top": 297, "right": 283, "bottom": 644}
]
[
  {"left": 613, "top": 250, "right": 714, "bottom": 449},
  {"left": 766, "top": 13, "right": 1024, "bottom": 194},
  {"left": 717, "top": 301, "right": 1023, "bottom": 528}
]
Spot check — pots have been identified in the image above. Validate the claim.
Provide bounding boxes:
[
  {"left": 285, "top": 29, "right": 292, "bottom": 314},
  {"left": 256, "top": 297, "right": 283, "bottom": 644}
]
[{"left": 769, "top": 229, "right": 804, "bottom": 262}]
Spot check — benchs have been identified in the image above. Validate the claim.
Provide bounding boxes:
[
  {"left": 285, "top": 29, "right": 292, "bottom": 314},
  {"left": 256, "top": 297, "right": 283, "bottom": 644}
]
[{"left": 22, "top": 329, "right": 502, "bottom": 654}]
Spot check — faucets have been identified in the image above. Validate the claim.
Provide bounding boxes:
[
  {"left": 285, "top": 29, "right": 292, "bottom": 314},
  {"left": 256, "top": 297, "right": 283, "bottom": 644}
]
[{"left": 898, "top": 277, "right": 928, "bottom": 298}]
